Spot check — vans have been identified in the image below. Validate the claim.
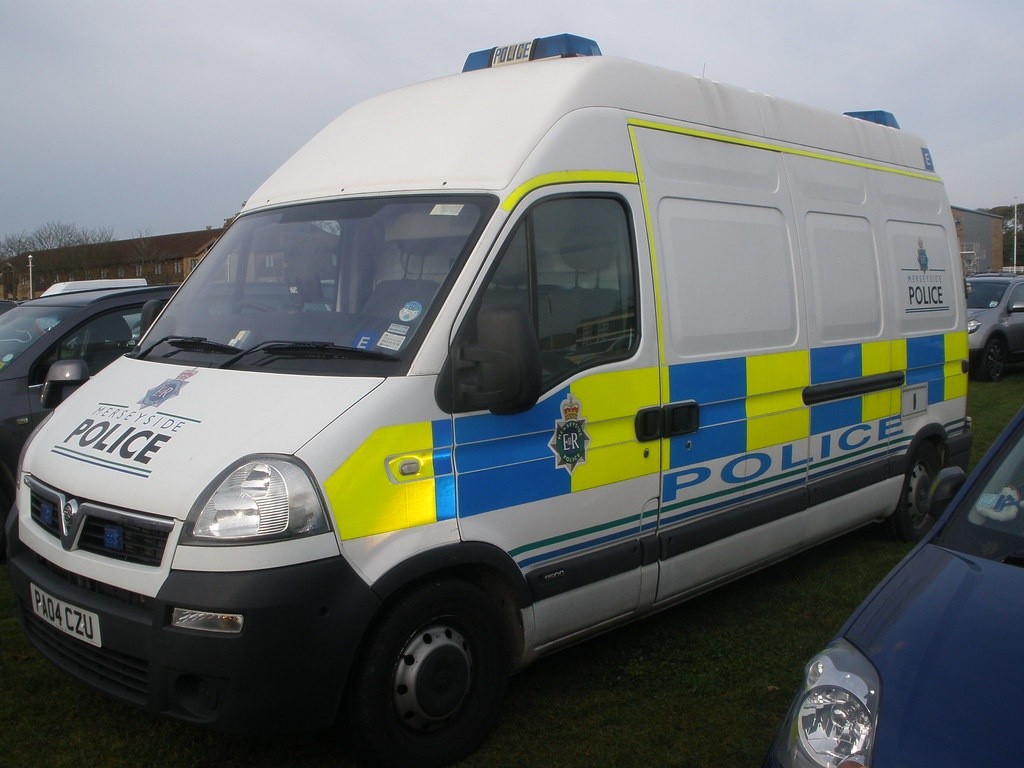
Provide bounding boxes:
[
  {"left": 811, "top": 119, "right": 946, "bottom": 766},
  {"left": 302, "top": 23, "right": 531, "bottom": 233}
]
[
  {"left": 34, "top": 277, "right": 150, "bottom": 343},
  {"left": 5, "top": 32, "right": 973, "bottom": 768}
]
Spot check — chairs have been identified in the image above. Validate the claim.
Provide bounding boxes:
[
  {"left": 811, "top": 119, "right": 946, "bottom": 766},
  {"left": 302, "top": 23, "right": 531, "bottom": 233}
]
[
  {"left": 557, "top": 227, "right": 635, "bottom": 345},
  {"left": 84, "top": 316, "right": 133, "bottom": 376},
  {"left": 469, "top": 240, "right": 550, "bottom": 354},
  {"left": 358, "top": 235, "right": 447, "bottom": 342}
]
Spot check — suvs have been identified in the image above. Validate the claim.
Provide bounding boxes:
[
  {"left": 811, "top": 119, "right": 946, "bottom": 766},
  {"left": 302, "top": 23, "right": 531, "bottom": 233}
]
[{"left": 963, "top": 271, "right": 1023, "bottom": 384}]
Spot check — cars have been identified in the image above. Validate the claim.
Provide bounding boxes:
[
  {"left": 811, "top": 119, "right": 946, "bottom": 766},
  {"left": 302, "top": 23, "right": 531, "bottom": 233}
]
[
  {"left": 757, "top": 400, "right": 1024, "bottom": 768},
  {"left": 0, "top": 283, "right": 336, "bottom": 511}
]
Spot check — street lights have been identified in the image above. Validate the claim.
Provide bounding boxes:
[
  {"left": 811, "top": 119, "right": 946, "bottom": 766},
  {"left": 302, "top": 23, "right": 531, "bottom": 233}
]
[
  {"left": 28, "top": 254, "right": 34, "bottom": 299},
  {"left": 1013, "top": 195, "right": 1019, "bottom": 273}
]
